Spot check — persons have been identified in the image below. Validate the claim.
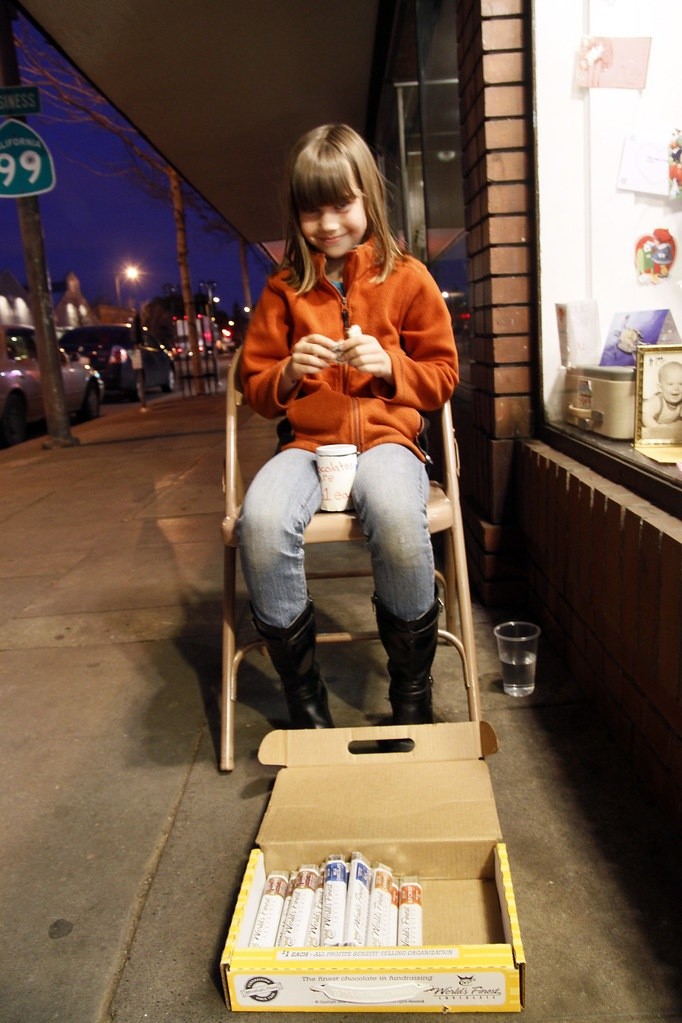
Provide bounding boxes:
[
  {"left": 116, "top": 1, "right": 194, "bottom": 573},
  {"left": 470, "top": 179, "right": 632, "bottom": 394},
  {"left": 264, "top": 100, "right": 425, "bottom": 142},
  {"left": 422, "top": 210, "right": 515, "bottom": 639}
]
[{"left": 235, "top": 122, "right": 458, "bottom": 727}]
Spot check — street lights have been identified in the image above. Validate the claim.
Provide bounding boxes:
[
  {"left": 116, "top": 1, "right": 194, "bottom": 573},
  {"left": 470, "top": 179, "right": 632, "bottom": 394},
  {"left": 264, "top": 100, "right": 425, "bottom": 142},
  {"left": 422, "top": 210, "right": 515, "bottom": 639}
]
[{"left": 115, "top": 265, "right": 139, "bottom": 322}]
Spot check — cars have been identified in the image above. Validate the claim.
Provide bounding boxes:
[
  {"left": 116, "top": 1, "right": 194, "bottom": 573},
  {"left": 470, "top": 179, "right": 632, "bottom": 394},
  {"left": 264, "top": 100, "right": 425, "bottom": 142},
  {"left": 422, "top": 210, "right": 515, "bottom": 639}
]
[
  {"left": 176, "top": 335, "right": 215, "bottom": 358},
  {"left": 62, "top": 322, "right": 175, "bottom": 400},
  {"left": 0, "top": 324, "right": 104, "bottom": 450}
]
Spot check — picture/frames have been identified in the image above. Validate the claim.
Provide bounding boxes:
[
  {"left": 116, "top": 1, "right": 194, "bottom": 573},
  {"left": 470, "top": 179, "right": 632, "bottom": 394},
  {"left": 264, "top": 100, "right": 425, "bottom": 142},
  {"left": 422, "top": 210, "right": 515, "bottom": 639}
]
[{"left": 633, "top": 345, "right": 682, "bottom": 448}]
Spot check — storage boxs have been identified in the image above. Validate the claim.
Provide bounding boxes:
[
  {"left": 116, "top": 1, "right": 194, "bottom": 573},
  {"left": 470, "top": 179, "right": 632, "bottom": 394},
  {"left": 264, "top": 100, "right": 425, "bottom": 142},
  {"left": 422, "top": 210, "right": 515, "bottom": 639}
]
[{"left": 220, "top": 721, "right": 527, "bottom": 1015}]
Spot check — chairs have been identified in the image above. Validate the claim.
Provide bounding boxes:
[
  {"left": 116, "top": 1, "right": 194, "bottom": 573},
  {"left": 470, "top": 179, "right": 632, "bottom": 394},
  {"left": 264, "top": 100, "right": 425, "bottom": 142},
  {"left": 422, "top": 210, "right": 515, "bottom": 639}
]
[{"left": 218, "top": 340, "right": 482, "bottom": 774}]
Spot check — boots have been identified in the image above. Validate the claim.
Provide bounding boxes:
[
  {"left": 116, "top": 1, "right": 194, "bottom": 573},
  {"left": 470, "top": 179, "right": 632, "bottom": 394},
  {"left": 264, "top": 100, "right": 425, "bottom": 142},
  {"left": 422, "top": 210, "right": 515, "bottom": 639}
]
[
  {"left": 371, "top": 582, "right": 444, "bottom": 753},
  {"left": 249, "top": 589, "right": 334, "bottom": 731}
]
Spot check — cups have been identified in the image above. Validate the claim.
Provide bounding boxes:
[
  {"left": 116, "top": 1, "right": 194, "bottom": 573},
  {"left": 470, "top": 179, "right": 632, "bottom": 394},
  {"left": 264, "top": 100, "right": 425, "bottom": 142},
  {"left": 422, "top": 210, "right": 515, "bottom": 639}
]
[
  {"left": 493, "top": 621, "right": 541, "bottom": 696},
  {"left": 315, "top": 444, "right": 357, "bottom": 512}
]
